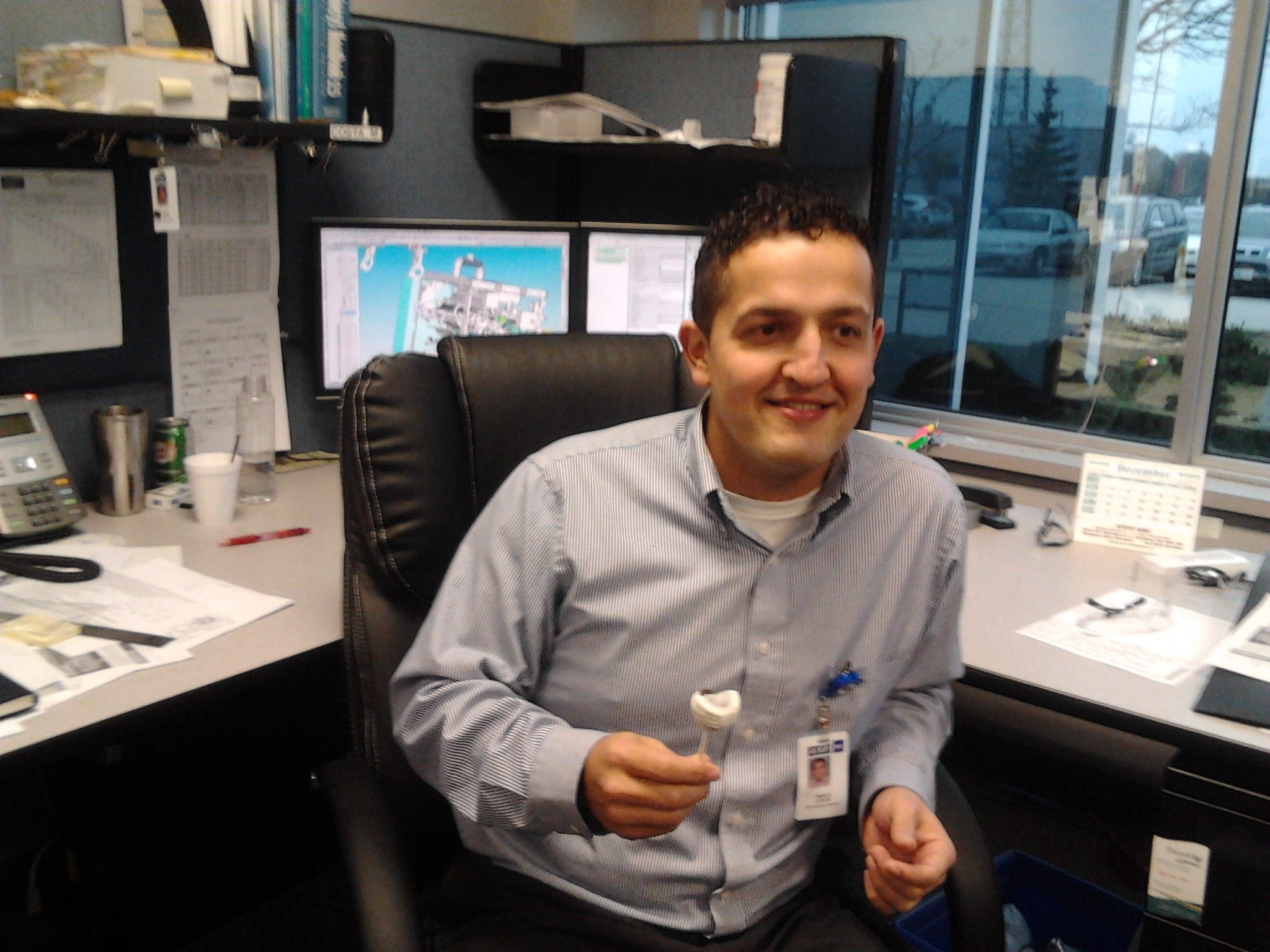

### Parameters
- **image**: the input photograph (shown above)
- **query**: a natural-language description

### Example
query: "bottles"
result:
[236,374,277,507]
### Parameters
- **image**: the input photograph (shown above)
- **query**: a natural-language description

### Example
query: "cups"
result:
[183,453,243,527]
[93,403,149,518]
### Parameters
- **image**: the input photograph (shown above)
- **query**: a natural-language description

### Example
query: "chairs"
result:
[330,336,1005,952]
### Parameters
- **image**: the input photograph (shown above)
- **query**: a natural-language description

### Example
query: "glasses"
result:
[1037,509,1073,545]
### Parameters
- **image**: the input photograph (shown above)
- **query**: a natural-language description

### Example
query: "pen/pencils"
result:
[218,527,309,546]
[905,418,942,455]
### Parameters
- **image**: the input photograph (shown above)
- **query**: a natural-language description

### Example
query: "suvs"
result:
[1071,194,1188,287]
[1230,204,1270,294]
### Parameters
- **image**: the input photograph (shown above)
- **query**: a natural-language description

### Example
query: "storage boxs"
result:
[895,849,1144,952]
[14,51,230,120]
[143,480,194,510]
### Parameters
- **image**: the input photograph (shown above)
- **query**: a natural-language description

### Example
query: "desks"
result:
[0,458,1270,952]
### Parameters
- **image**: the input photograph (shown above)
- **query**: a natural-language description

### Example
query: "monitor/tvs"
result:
[580,221,706,352]
[310,217,580,399]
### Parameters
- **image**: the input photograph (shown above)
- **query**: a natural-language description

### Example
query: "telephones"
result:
[0,394,89,539]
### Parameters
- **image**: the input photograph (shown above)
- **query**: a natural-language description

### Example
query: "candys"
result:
[691,687,743,731]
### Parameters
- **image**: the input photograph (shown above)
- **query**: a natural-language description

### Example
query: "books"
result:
[122,1,316,123]
[0,675,38,722]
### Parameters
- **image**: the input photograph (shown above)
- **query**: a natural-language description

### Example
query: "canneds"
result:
[151,416,194,488]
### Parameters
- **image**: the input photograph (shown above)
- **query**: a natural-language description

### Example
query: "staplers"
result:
[957,482,1014,530]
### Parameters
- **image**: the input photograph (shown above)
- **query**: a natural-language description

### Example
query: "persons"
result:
[807,757,829,787]
[388,174,970,952]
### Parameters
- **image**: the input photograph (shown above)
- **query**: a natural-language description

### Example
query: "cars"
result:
[1184,204,1206,274]
[889,191,955,238]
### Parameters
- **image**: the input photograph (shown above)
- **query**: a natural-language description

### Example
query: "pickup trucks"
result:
[974,206,1090,277]
